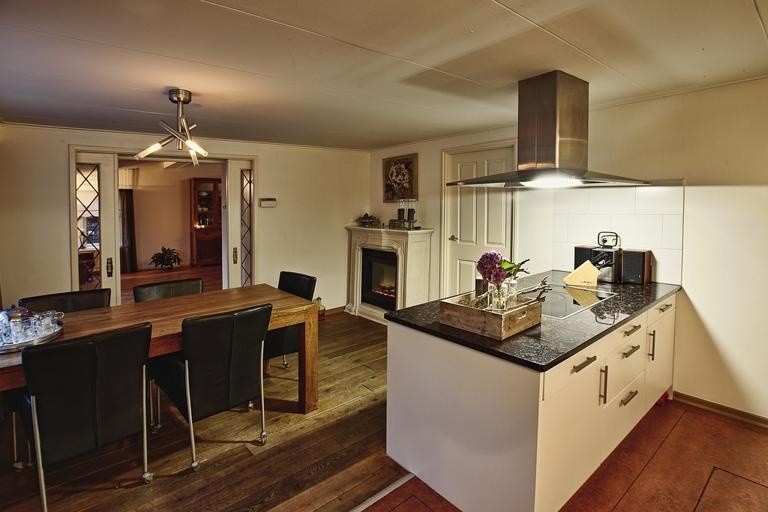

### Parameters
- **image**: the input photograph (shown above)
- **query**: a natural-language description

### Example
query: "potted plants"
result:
[148,246,181,270]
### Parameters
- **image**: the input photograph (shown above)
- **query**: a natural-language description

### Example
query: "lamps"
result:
[134,89,208,168]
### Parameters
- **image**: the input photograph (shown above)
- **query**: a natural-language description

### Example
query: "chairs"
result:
[147,302,273,473]
[133,277,203,429]
[18,287,112,467]
[12,320,153,511]
[265,271,318,371]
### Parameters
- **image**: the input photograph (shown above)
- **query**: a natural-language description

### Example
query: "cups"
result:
[0,309,65,345]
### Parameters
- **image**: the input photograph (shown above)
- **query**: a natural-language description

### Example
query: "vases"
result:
[487,280,518,314]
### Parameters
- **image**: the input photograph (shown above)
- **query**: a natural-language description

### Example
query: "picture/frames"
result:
[381,152,422,203]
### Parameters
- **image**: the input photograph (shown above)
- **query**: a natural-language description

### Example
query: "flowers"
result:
[477,253,530,307]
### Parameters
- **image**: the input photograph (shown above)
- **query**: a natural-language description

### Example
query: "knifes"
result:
[590,251,612,270]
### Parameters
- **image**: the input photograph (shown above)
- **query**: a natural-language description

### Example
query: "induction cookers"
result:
[515,282,619,320]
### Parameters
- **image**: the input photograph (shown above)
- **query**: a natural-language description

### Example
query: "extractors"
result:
[446,70,653,190]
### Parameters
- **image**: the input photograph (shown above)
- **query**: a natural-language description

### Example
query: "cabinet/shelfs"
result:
[78,251,96,283]
[536,292,676,512]
[190,177,223,266]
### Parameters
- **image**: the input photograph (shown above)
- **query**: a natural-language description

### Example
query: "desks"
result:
[2,282,319,416]
